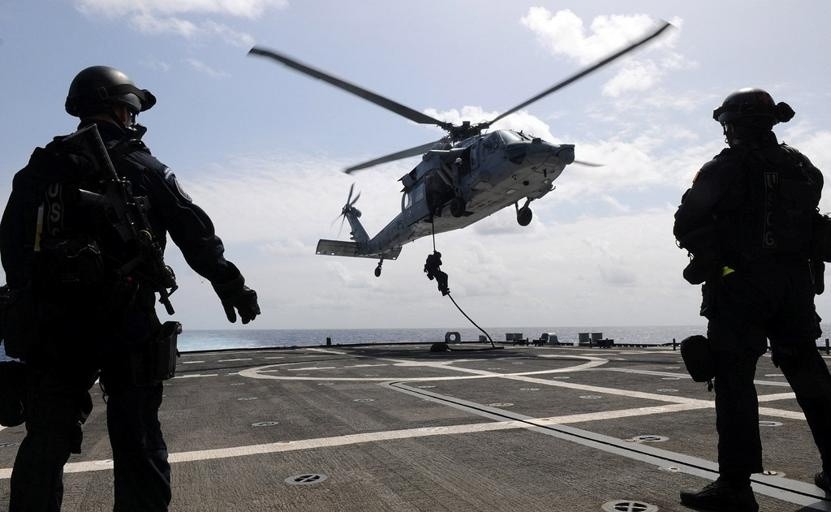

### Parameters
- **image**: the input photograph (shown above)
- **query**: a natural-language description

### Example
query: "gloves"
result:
[219,277,260,325]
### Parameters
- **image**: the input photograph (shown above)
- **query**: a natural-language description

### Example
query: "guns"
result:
[61,123,178,315]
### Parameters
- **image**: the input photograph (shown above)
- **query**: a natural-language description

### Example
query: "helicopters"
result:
[239,18,680,284]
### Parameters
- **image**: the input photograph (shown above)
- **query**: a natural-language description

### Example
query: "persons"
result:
[0,65,261,512]
[426,251,450,296]
[673,88,830,512]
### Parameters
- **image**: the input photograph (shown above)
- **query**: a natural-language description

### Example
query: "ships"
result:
[1,335,831,512]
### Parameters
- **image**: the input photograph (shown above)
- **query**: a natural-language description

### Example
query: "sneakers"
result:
[679,472,758,512]
[439,285,451,296]
[423,218,433,224]
[433,211,442,218]
[814,469,830,491]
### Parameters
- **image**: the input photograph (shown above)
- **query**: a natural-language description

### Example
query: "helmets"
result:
[65,65,144,117]
[712,89,776,121]
